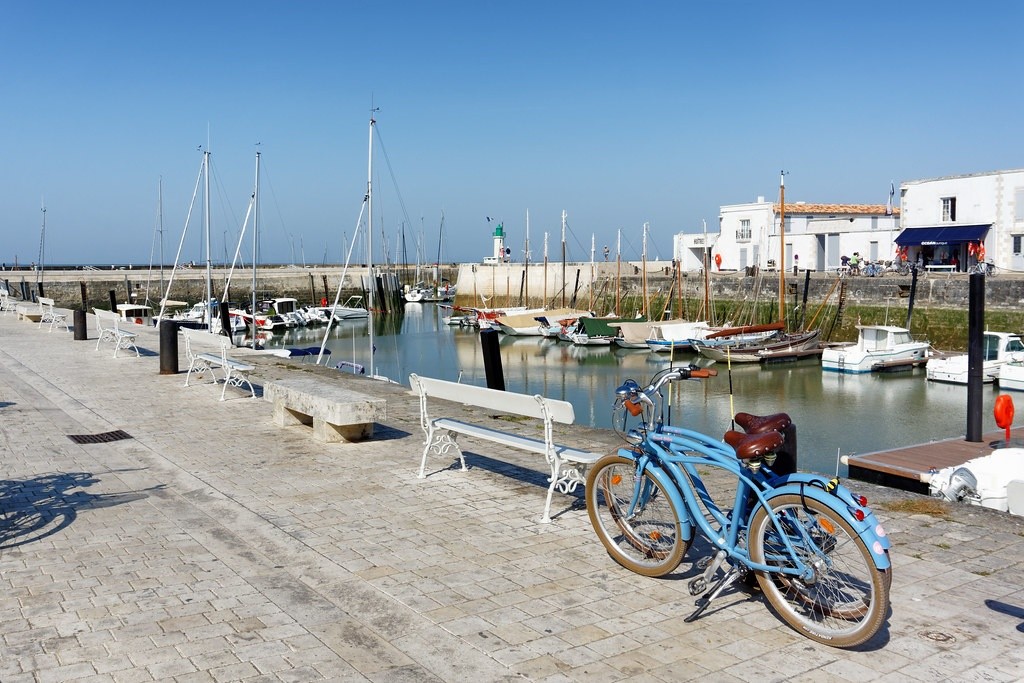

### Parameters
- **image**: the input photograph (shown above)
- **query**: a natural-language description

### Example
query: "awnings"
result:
[894,224,993,246]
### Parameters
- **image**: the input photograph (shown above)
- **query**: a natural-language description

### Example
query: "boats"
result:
[821,326,931,373]
[228,296,344,330]
[924,331,1024,384]
[316,295,368,319]
[91,303,155,329]
[998,356,1024,391]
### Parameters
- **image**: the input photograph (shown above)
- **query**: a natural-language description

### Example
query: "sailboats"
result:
[141,122,247,347]
[687,166,844,365]
[314,92,408,384]
[394,209,456,302]
[445,205,759,354]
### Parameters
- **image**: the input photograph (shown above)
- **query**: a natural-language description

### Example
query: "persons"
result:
[917,250,923,267]
[604,245,609,262]
[500,246,511,263]
[850,252,858,275]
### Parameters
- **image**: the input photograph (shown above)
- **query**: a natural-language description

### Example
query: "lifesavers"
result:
[474,324,479,328]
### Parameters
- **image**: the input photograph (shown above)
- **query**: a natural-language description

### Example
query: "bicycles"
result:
[585,361,894,648]
[859,260,884,277]
[967,259,1000,278]
[897,259,926,276]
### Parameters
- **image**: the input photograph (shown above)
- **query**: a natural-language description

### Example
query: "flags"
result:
[886,182,894,215]
[486,216,494,222]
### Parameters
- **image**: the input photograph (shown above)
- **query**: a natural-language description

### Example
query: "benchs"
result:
[92,306,141,358]
[824,266,850,279]
[179,326,256,402]
[36,295,69,333]
[408,372,606,523]
[15,304,42,322]
[0,289,18,316]
[263,378,387,443]
[924,265,957,280]
